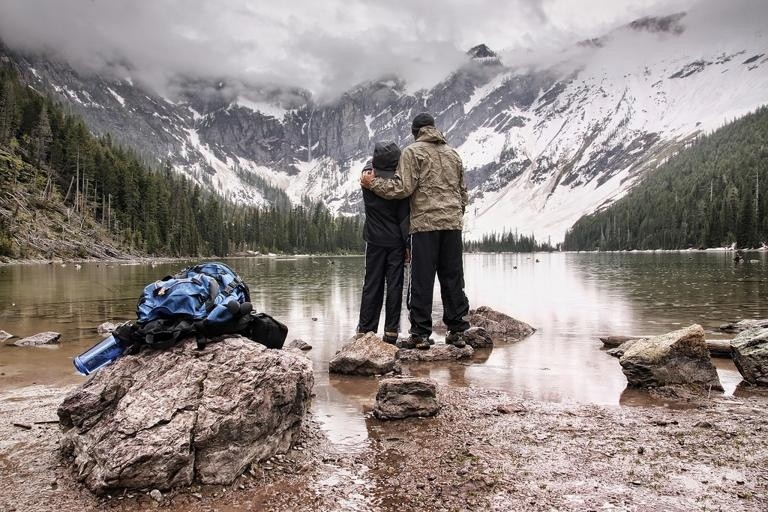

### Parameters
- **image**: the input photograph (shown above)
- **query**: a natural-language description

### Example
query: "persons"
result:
[354,140,412,344]
[360,113,469,349]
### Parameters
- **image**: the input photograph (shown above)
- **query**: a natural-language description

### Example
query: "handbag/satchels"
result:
[247,311,287,349]
[134,263,252,348]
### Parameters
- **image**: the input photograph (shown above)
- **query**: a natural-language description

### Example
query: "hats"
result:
[411,113,434,136]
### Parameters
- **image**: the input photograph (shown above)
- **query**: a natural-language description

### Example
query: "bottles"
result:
[74,334,124,376]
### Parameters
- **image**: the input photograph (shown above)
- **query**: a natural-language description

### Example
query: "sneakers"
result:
[406,334,431,349]
[383,329,398,343]
[356,323,375,335]
[445,329,466,347]
